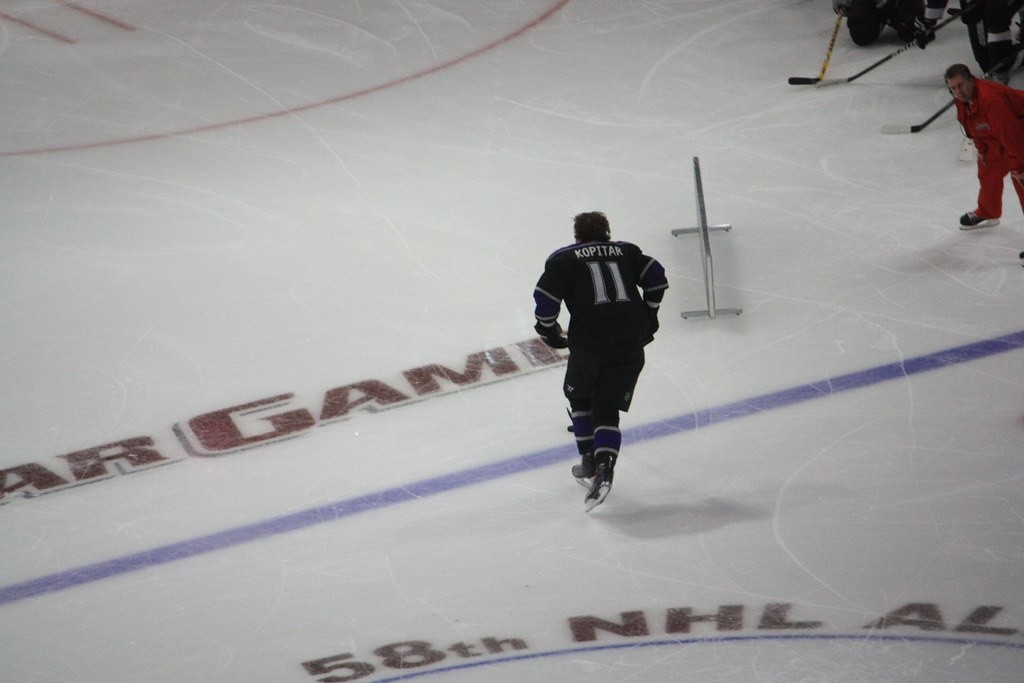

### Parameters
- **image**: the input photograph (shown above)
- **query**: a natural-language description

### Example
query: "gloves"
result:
[914,16,936,49]
[832,0,852,17]
[644,304,659,335]
[534,321,568,349]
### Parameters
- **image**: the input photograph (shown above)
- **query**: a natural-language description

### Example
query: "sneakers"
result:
[959,211,1000,230]
[572,459,596,488]
[584,455,614,512]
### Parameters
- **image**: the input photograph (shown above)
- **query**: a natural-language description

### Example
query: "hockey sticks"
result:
[788,5,961,89]
[880,61,1004,136]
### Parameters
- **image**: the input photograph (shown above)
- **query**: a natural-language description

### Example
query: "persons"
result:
[532,212,669,512]
[830,0,1024,265]
